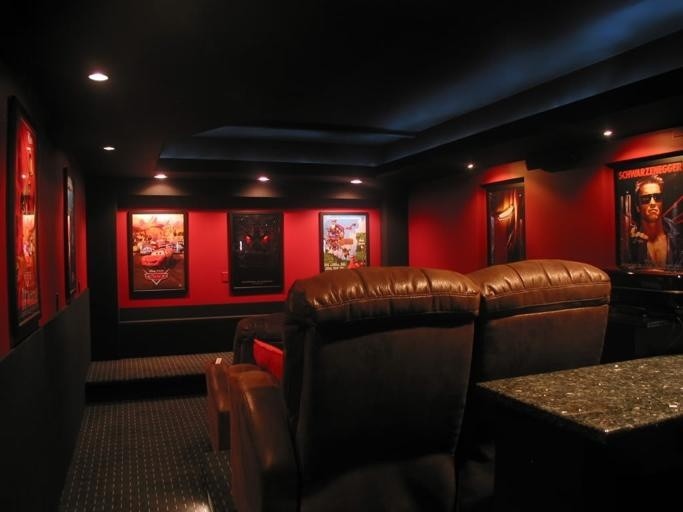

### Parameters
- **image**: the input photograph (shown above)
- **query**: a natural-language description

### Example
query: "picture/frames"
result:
[126,210,189,298]
[225,210,285,295]
[317,211,370,273]
[5,97,42,327]
[62,166,76,299]
[604,149,683,272]
[479,176,525,267]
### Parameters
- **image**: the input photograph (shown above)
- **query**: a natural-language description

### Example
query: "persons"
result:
[326,217,345,251]
[624,174,683,269]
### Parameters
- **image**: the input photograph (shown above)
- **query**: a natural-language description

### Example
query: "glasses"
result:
[635,193,666,205]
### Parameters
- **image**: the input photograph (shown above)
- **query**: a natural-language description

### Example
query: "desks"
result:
[477,339,682,512]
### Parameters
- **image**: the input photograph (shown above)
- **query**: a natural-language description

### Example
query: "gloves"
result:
[629,235,648,261]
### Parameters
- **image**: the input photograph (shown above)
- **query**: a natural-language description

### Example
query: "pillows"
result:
[251,337,286,376]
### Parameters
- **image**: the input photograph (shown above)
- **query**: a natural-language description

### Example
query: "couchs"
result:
[469,261,611,374]
[202,267,480,512]
[202,313,282,451]
